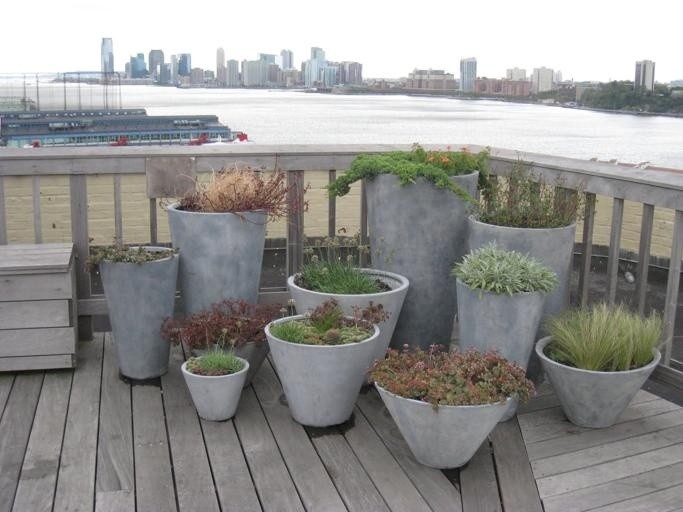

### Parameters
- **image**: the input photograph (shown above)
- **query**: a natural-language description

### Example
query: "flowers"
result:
[187,328,249,373]
[264,298,393,345]
[366,342,536,407]
[297,226,396,295]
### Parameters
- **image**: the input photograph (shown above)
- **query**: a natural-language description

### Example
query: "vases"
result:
[360,382,537,472]
[181,357,249,421]
[364,170,480,354]
[265,314,380,427]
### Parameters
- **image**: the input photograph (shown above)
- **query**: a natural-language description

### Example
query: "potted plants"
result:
[286,268,409,395]
[322,142,499,207]
[166,150,312,341]
[85,236,180,381]
[160,297,284,390]
[533,298,667,430]
[464,151,599,378]
[449,242,558,422]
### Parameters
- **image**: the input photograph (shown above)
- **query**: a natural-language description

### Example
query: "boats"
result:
[1,71,243,147]
